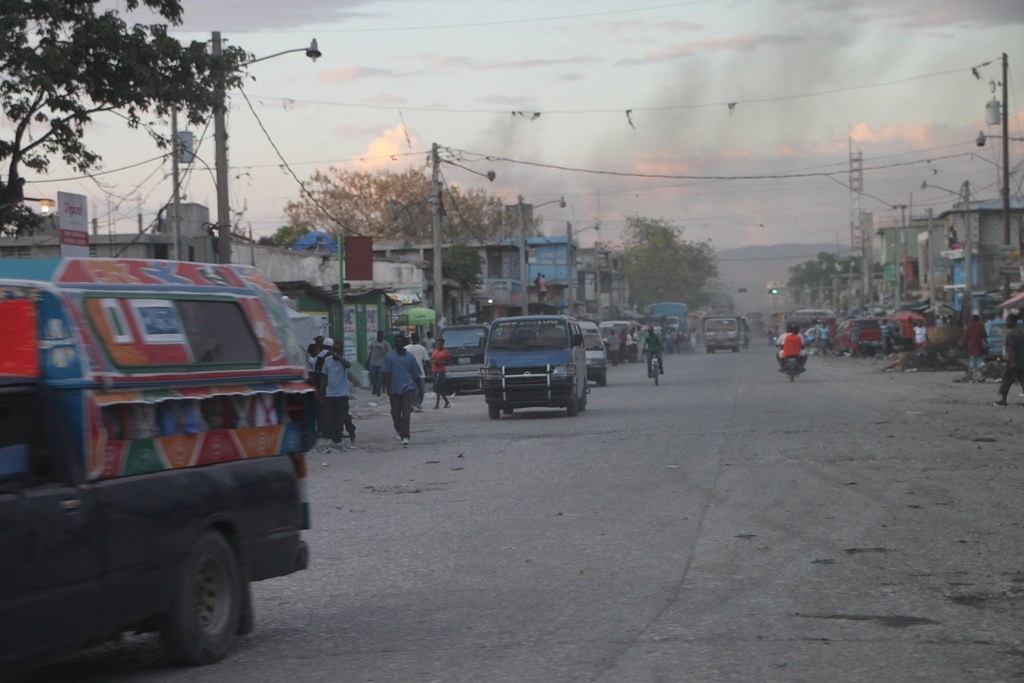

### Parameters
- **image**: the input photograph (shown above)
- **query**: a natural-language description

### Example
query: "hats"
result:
[647,326,653,331]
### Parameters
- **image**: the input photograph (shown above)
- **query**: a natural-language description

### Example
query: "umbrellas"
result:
[392,305,436,337]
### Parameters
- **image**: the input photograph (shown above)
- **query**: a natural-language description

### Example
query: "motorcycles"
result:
[773,336,808,380]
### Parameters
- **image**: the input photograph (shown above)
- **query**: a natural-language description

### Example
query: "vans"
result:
[573,320,610,387]
[598,302,688,366]
[597,320,647,363]
[434,319,492,396]
[765,304,1024,378]
[479,313,589,420]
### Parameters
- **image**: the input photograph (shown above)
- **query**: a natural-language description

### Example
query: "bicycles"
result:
[641,347,665,386]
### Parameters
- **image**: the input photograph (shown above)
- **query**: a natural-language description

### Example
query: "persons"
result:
[381,336,424,445]
[903,316,927,351]
[993,314,1024,408]
[430,339,452,409]
[964,315,988,369]
[366,330,392,396]
[606,326,644,366]
[306,336,356,447]
[400,332,435,413]
[805,319,860,357]
[534,272,548,302]
[879,319,891,352]
[664,327,699,354]
[436,313,447,329]
[935,314,952,325]
[776,324,808,372]
[643,326,664,378]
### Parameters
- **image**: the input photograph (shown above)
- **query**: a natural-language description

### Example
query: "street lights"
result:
[919,177,971,323]
[212,31,323,265]
[517,192,567,315]
[565,220,601,317]
[431,141,497,341]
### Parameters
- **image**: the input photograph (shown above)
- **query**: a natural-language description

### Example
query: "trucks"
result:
[703,315,750,354]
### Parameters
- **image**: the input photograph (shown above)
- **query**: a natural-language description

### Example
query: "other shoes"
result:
[331,442,337,447]
[396,432,401,439]
[403,437,408,445]
[350,433,355,441]
[993,400,1006,408]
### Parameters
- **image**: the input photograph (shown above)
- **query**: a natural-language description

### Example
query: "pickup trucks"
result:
[0,370,314,683]
